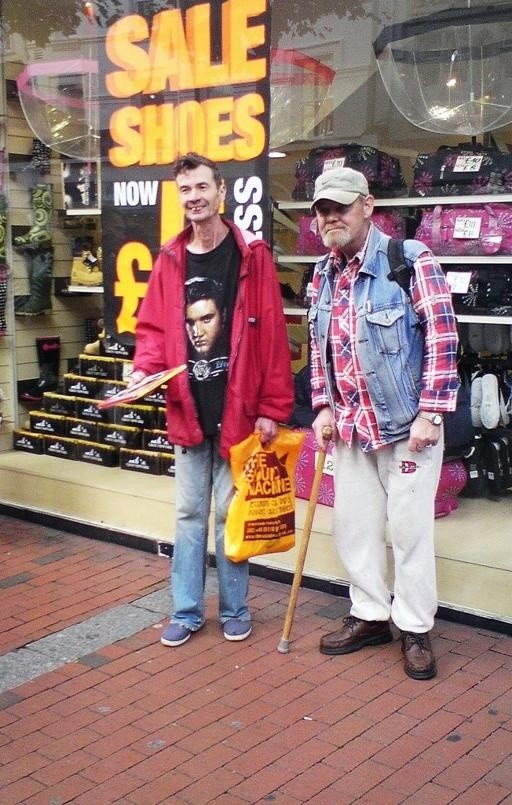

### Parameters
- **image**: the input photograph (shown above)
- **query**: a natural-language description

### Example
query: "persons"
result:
[309,168,459,679]
[183,276,226,355]
[127,153,296,646]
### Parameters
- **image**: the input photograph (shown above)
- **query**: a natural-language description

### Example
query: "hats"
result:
[310,167,369,212]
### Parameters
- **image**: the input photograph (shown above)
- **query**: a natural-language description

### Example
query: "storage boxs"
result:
[12,338,177,478]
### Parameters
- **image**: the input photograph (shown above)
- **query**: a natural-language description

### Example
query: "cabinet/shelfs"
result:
[64,134,512,326]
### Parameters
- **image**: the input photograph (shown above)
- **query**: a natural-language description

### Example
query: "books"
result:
[97,365,188,411]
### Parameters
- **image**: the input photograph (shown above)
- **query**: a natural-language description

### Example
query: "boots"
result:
[20,137,52,176]
[84,328,105,355]
[15,246,55,318]
[72,258,103,287]
[23,336,61,400]
[13,184,54,245]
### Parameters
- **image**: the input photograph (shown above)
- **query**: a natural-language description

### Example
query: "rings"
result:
[425,444,432,448]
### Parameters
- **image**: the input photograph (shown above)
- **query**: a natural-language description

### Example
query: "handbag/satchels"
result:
[452,274,511,316]
[296,208,406,257]
[443,379,473,454]
[292,142,408,201]
[410,141,511,198]
[411,204,511,256]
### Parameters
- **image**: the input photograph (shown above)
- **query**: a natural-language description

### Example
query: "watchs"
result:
[417,411,444,425]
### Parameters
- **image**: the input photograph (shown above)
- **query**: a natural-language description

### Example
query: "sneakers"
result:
[161,623,192,647]
[460,364,512,496]
[224,618,250,642]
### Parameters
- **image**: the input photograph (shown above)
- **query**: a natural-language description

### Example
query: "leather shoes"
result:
[400,630,438,680]
[320,615,394,655]
